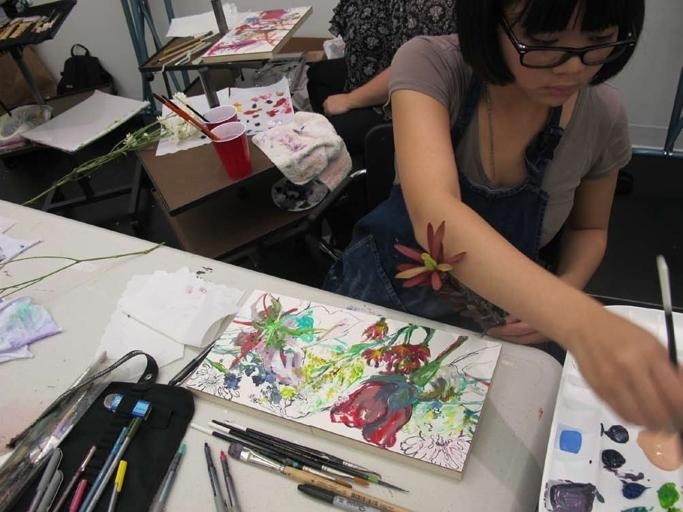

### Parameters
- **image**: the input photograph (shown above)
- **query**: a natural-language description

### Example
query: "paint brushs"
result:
[191,417,413,512]
[57,349,107,408]
[151,30,215,66]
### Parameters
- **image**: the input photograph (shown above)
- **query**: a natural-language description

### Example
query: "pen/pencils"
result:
[148,93,217,141]
[167,340,217,386]
[654,254,679,362]
[29,416,147,511]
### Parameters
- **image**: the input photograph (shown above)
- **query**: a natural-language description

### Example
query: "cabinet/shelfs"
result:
[0,0,312,262]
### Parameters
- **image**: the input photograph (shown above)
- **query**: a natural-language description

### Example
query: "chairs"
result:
[304,124,396,289]
[534,147,683,363]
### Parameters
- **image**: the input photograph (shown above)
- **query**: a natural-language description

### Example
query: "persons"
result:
[339,0,683,433]
[306,0,461,138]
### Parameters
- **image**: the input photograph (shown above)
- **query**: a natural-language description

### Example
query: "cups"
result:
[201,104,238,131]
[207,121,253,182]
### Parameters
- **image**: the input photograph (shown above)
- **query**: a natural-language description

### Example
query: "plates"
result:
[270,174,328,212]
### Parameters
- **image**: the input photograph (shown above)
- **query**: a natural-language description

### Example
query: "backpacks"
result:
[57,44,118,95]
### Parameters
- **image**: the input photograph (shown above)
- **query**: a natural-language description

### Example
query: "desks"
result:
[0,201,565,511]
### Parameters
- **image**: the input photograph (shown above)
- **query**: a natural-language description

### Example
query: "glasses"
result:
[499,13,637,69]
